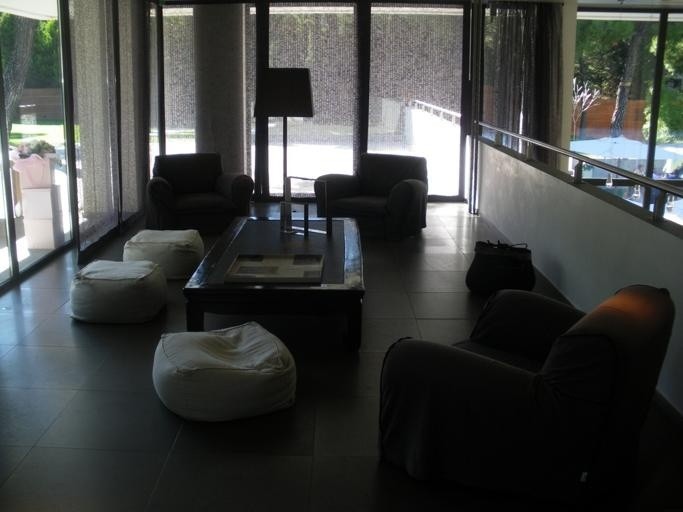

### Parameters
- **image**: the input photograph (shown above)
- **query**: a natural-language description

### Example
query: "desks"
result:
[180,216,366,370]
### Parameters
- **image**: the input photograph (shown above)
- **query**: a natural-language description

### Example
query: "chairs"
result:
[375,281,677,512]
[314,151,429,242]
[143,151,255,231]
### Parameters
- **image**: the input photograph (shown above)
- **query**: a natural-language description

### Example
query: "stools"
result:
[148,320,298,423]
[122,227,206,282]
[68,256,168,325]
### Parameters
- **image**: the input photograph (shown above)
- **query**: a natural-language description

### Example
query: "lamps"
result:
[252,67,316,221]
